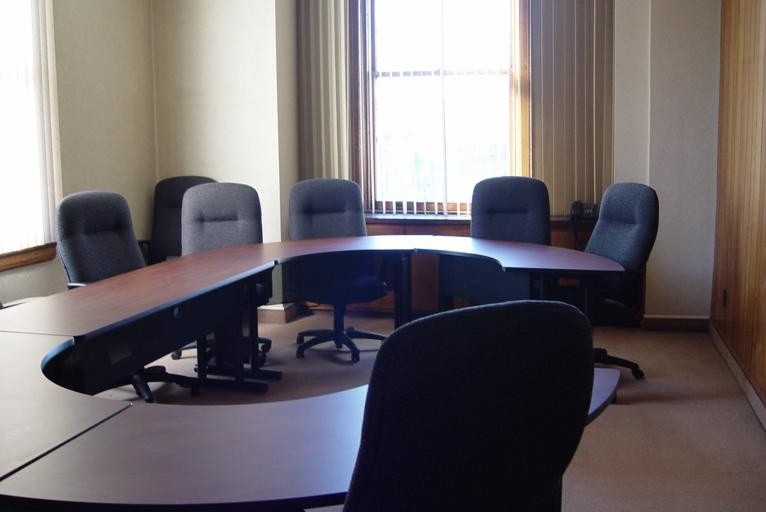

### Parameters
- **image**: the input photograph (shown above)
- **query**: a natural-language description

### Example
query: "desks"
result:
[0,231,630,512]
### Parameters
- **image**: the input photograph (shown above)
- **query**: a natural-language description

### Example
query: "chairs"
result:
[279,174,394,366]
[327,300,590,512]
[555,178,661,385]
[51,186,208,401]
[144,174,226,363]
[165,179,281,386]
[470,173,557,249]
